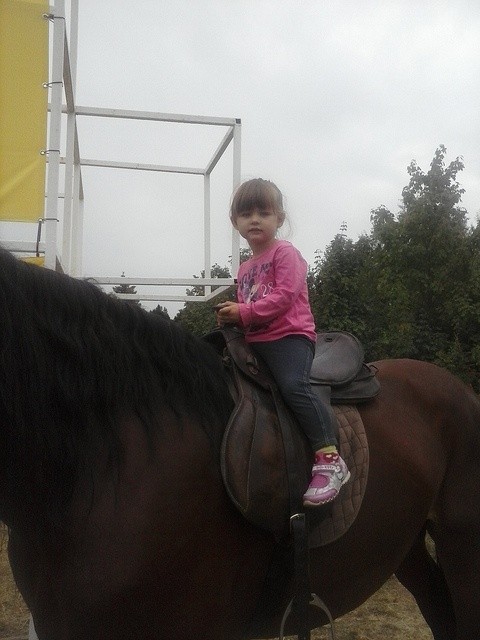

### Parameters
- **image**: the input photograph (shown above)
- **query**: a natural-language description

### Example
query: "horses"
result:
[0,249,480,640]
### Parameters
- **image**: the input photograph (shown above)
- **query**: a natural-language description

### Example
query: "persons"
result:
[214,179,352,507]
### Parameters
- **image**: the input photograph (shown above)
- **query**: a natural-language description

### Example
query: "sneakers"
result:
[301,454,353,509]
